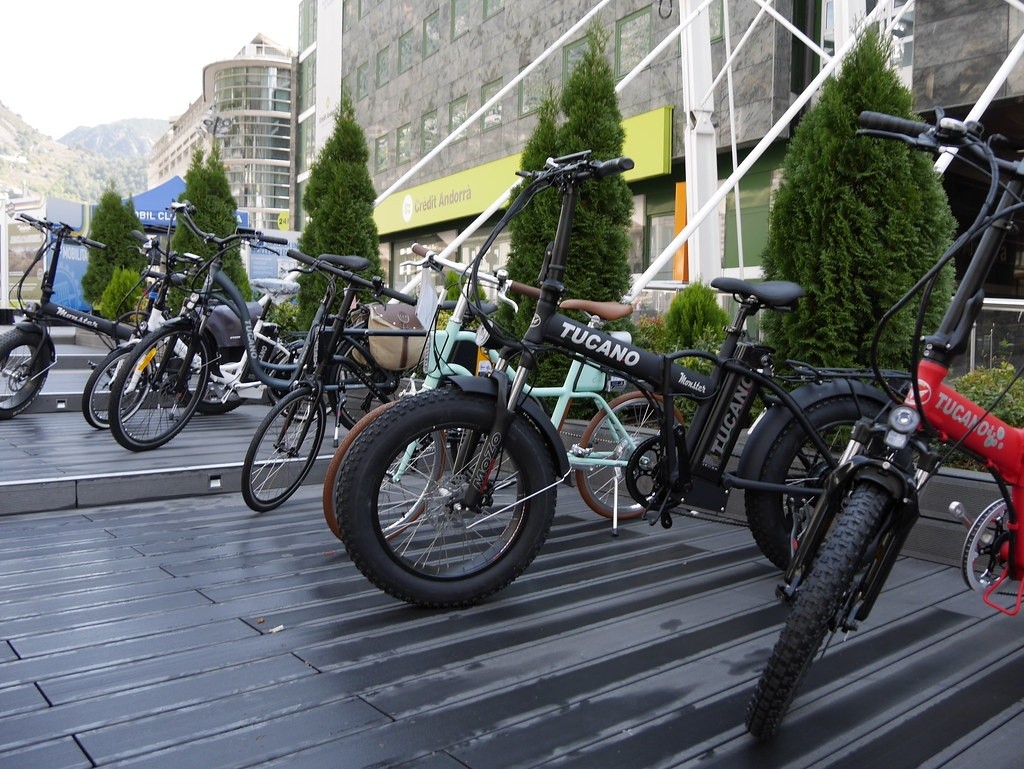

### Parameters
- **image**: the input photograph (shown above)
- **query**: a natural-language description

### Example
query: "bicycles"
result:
[0,199,549,452]
[333,150,911,609]
[242,248,548,513]
[322,243,686,542]
[744,109,1024,740]
